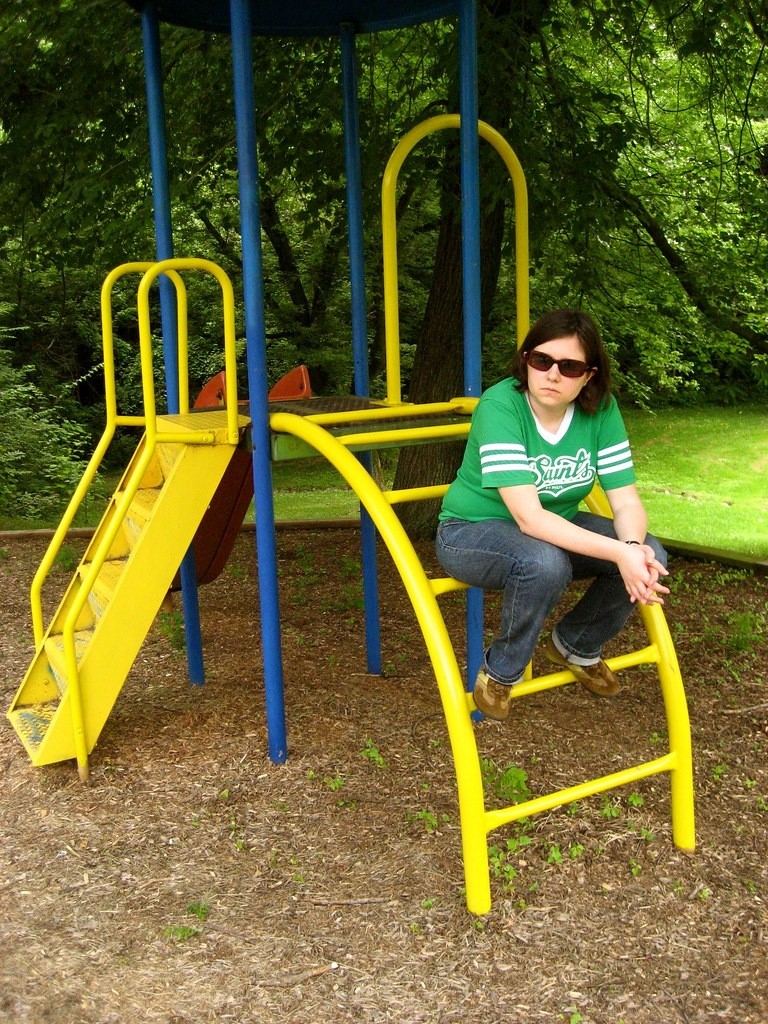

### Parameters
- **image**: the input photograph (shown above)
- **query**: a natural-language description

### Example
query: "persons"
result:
[437,309,670,721]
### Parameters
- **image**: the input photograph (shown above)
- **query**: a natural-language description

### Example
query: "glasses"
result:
[526,348,590,377]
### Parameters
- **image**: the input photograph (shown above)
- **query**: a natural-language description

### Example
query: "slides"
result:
[168,364,312,593]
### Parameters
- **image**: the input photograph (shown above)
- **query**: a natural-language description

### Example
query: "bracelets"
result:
[625,541,640,546]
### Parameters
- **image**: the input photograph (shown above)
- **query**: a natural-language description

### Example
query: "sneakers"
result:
[472,658,511,721]
[540,631,622,699]
